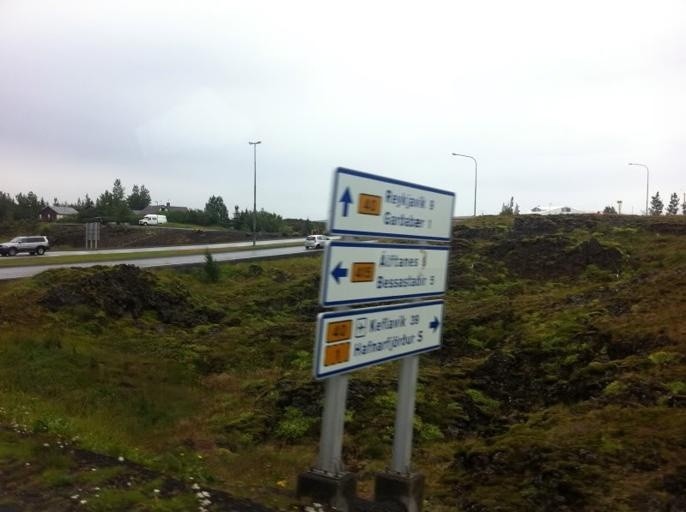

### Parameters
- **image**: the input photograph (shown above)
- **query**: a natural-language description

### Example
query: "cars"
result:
[0,235,50,256]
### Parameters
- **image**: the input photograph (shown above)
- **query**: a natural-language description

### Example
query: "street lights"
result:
[452,153,478,216]
[628,163,649,215]
[248,139,261,245]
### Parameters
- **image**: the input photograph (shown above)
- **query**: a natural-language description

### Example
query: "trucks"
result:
[139,214,167,226]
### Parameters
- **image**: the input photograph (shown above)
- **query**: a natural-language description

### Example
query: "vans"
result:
[304,235,330,249]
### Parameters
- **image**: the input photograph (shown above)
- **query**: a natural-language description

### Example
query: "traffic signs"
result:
[314,167,456,381]
